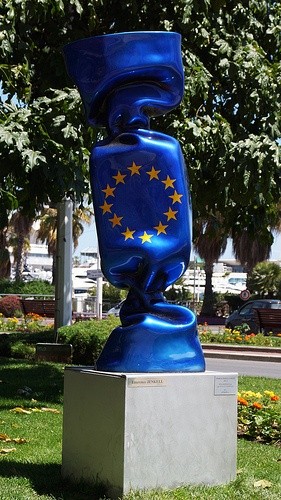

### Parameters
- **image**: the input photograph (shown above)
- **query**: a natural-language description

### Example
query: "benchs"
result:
[17,299,56,317]
[251,307,281,335]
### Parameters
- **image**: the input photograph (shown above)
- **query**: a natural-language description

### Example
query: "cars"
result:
[224,299,281,330]
[106,299,127,316]
